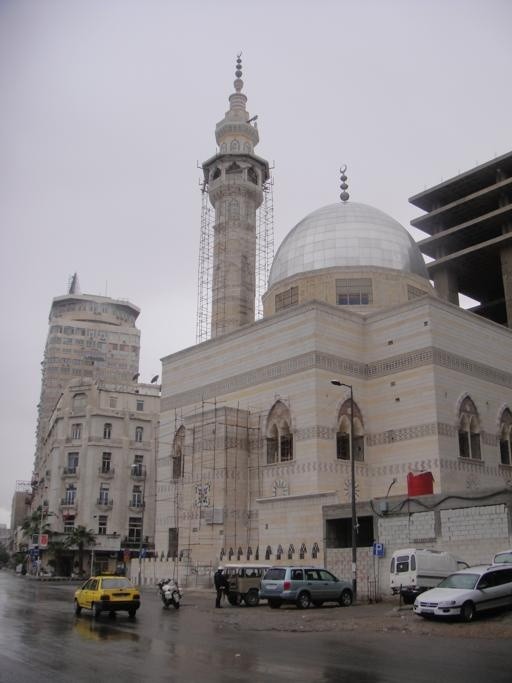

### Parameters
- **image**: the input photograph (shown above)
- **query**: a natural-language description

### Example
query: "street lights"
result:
[330,379,359,601]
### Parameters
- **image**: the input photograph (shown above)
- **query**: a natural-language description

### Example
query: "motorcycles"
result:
[158,578,181,608]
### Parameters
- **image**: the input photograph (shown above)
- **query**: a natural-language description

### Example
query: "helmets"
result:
[217,566,224,569]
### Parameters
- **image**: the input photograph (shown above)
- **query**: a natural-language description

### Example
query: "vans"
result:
[390,547,472,603]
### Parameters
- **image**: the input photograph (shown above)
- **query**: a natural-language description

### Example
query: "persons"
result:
[214,566,225,608]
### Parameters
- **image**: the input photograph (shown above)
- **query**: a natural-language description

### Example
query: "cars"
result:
[74,575,141,617]
[412,552,511,622]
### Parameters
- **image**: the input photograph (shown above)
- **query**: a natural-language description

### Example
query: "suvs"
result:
[223,564,273,606]
[260,565,355,608]
